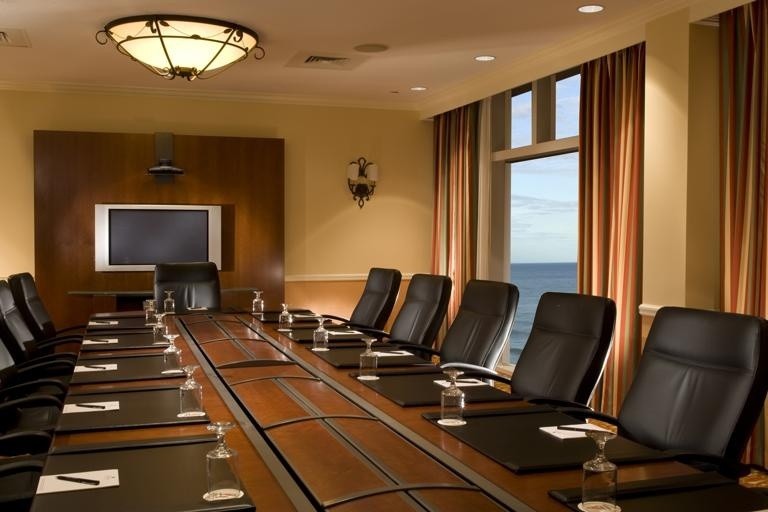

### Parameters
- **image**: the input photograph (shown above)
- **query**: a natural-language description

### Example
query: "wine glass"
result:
[580,429,617,511]
[145,299,204,414]
[205,422,241,497]
[439,368,466,423]
[279,303,293,330]
[162,290,175,313]
[311,317,330,349]
[359,337,379,380]
[252,290,265,314]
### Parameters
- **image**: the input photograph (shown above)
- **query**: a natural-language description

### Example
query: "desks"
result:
[30,307,768,511]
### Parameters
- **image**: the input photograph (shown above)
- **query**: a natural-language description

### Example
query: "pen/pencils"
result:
[380,351,404,354]
[57,476,99,485]
[85,366,106,369]
[557,426,615,435]
[447,380,478,383]
[76,404,105,409]
[91,322,109,342]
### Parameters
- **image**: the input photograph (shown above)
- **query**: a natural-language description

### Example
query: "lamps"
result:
[346,157,378,211]
[95,14,265,82]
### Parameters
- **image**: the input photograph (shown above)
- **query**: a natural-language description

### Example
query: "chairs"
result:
[0,269,89,511]
[320,268,403,337]
[551,305,768,461]
[392,279,521,389]
[458,292,616,413]
[154,262,222,313]
[353,274,452,364]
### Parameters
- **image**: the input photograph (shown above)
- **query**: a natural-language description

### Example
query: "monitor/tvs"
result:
[95,204,222,272]
